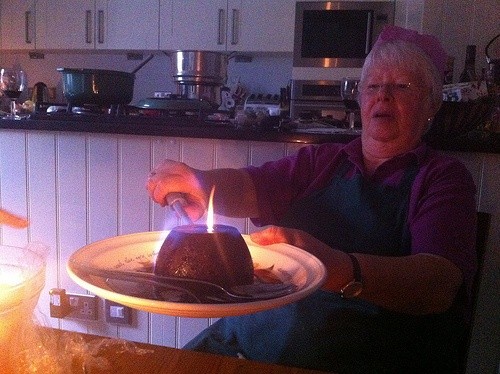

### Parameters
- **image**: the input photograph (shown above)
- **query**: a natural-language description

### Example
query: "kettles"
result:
[31,82,50,109]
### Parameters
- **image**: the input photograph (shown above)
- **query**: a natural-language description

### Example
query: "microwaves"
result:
[292,1,395,69]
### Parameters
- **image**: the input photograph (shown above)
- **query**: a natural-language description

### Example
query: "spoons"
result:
[75,262,299,298]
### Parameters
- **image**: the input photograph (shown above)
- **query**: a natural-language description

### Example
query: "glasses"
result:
[361,80,412,94]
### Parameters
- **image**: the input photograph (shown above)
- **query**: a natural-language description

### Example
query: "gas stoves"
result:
[30,108,235,127]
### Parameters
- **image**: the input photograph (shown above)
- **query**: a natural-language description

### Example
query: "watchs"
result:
[334,254,363,300]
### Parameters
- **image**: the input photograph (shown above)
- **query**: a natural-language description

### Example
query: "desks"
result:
[0,324,333,374]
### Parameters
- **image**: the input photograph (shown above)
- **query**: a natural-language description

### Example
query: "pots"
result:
[57,52,155,105]
[159,49,239,107]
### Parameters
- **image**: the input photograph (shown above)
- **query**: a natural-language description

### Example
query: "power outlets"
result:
[64,293,99,323]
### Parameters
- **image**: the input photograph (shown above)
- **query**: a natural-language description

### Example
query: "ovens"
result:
[288,79,360,129]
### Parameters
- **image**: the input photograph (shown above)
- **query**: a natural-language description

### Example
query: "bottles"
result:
[459,44,476,83]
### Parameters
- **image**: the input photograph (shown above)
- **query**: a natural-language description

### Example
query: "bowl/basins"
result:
[0,243,47,374]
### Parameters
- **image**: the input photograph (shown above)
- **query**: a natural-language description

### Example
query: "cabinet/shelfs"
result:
[392,0,500,71]
[0,0,298,58]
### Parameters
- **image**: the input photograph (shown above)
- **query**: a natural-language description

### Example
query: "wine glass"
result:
[339,77,362,135]
[0,68,30,121]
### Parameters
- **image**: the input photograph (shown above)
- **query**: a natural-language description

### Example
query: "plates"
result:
[67,230,328,318]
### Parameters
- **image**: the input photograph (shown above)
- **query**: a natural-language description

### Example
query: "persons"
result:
[145,25,479,374]
